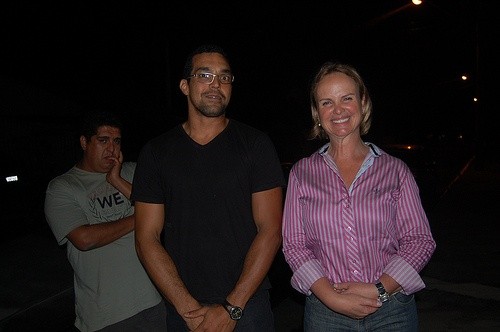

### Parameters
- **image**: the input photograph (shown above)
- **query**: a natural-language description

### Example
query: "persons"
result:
[45,109,168,332]
[129,44,287,332]
[282,62,436,332]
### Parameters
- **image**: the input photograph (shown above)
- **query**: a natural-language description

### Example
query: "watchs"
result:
[221,298,243,321]
[375,280,390,303]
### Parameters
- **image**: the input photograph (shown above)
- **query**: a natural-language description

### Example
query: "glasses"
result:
[187,72,235,84]
